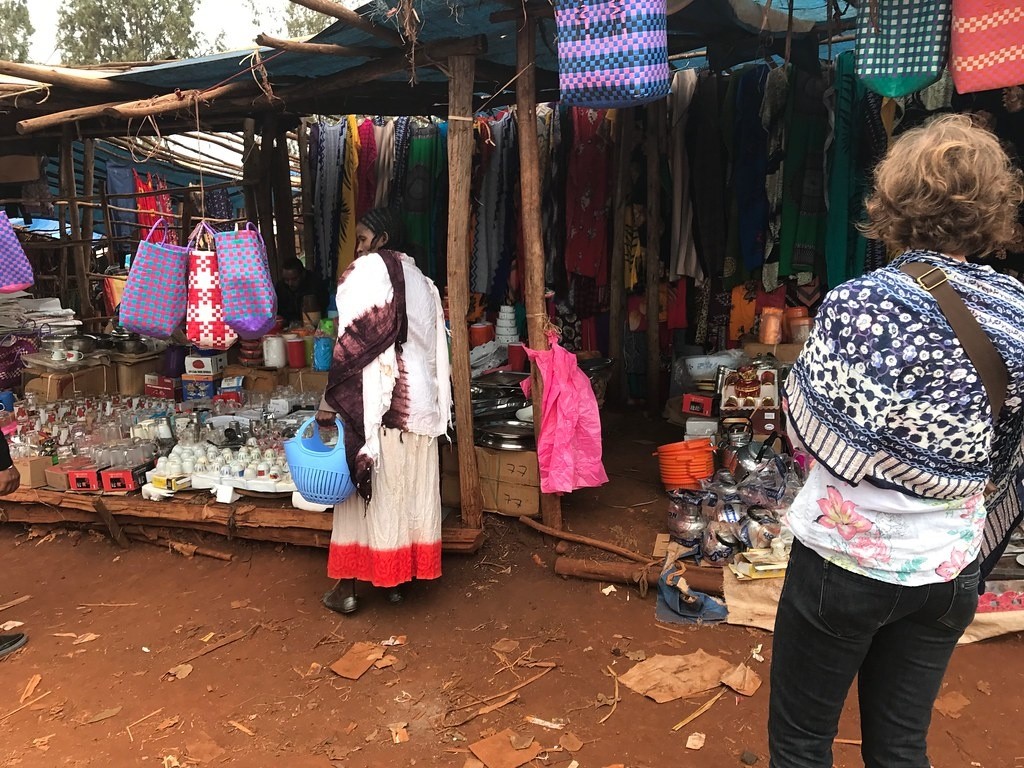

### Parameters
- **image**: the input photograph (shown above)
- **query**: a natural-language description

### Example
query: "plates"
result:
[469,341,501,367]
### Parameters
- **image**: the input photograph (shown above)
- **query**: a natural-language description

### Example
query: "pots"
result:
[113,333,148,355]
[89,331,113,349]
[63,330,96,353]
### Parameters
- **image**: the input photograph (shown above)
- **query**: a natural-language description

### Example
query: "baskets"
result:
[282,414,357,506]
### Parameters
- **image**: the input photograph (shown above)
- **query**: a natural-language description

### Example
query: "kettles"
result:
[749,351,793,379]
[314,337,333,371]
[665,416,805,566]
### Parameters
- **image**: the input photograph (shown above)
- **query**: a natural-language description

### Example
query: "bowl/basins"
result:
[683,354,739,381]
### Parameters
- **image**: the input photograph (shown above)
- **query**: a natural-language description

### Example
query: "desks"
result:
[0,484,483,553]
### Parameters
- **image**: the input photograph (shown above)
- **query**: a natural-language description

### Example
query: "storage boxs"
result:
[442,445,540,516]
[13,353,329,492]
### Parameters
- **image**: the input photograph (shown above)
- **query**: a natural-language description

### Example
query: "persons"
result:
[765,111,1024,767]
[314,208,455,615]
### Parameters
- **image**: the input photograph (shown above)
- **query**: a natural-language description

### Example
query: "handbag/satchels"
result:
[119,218,279,351]
[554,0,672,110]
[0,210,36,295]
[853,0,1024,98]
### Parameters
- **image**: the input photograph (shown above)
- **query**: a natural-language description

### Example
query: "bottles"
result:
[304,336,313,365]
[783,307,813,343]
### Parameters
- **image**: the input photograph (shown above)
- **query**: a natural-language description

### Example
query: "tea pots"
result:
[302,294,321,325]
[327,295,338,319]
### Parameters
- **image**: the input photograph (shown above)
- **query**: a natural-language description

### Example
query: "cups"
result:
[50,348,67,360]
[507,343,528,371]
[470,317,495,345]
[175,418,190,433]
[263,337,286,368]
[287,339,306,368]
[66,350,84,361]
[176,384,320,415]
[157,437,294,484]
[0,392,176,469]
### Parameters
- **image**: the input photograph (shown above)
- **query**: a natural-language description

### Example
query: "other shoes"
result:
[0,633,30,657]
[380,584,406,606]
[322,577,373,615]
